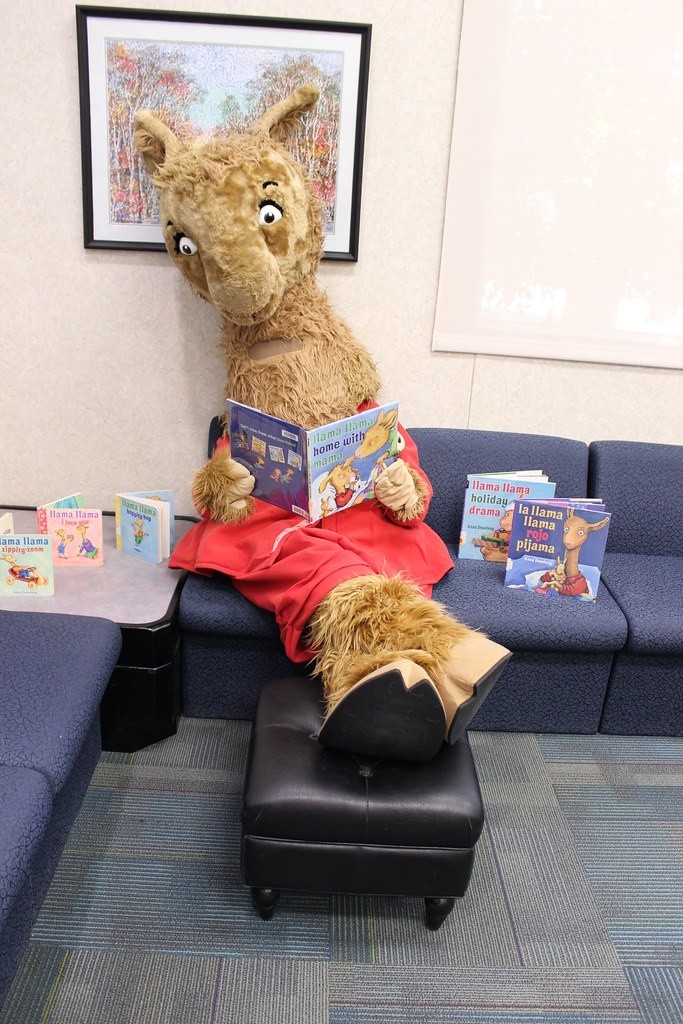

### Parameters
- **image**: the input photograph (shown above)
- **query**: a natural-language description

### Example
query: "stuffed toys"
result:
[133,85,510,762]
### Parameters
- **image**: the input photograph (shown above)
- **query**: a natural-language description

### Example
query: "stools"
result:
[238,676,485,931]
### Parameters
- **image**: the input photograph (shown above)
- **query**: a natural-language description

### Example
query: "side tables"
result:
[0,505,200,755]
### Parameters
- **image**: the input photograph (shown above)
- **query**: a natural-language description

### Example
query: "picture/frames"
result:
[76,4,373,263]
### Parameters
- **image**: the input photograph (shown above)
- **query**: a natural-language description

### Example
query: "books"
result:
[36,492,104,568]
[503,498,611,603]
[0,512,55,596]
[228,399,398,525]
[115,489,176,565]
[457,469,556,564]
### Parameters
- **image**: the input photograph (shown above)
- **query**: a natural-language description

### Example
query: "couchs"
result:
[180,414,682,739]
[1,610,121,930]
